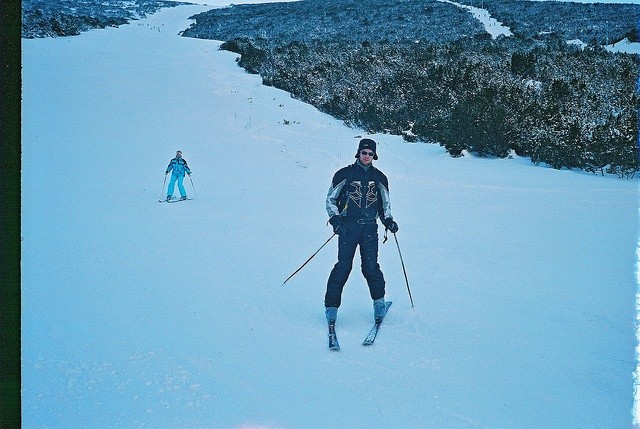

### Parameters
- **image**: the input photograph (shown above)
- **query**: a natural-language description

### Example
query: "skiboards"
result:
[158,196,193,203]
[328,302,393,350]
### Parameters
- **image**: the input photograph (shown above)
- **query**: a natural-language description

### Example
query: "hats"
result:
[176,151,182,158]
[355,139,378,160]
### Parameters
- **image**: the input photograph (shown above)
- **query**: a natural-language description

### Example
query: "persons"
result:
[164,151,191,203]
[324,139,398,327]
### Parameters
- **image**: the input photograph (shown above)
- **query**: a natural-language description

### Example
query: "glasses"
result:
[360,151,373,156]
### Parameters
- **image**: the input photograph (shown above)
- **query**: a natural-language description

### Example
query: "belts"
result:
[344,217,377,225]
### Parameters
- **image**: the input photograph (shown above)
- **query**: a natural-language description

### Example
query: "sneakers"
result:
[326,307,337,320]
[179,196,186,201]
[373,297,385,318]
[165,195,171,202]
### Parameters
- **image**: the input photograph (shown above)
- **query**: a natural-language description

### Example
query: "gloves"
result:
[165,171,170,175]
[326,215,344,235]
[188,173,192,177]
[382,218,398,233]
